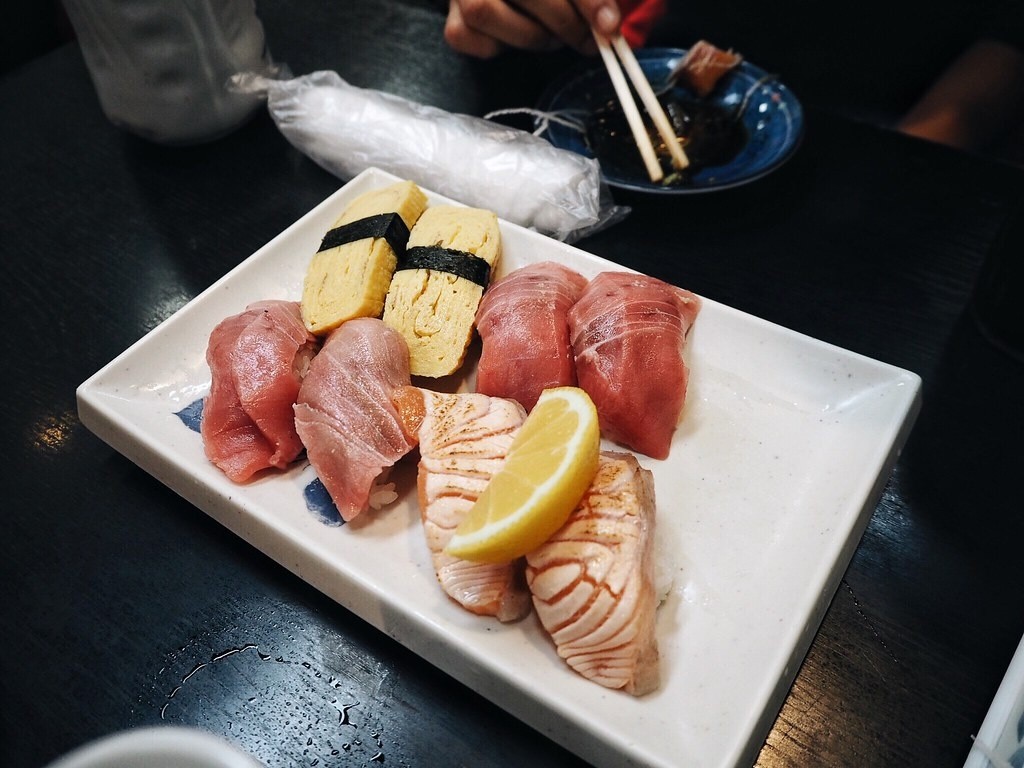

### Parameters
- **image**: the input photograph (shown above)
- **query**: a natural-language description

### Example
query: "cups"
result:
[63,0,278,146]
[36,724,263,768]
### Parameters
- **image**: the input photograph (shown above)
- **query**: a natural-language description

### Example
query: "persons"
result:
[442,0,1024,153]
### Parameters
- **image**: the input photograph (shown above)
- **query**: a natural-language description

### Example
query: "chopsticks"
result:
[592,20,691,182]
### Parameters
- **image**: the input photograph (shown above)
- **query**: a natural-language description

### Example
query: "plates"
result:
[70,166,923,768]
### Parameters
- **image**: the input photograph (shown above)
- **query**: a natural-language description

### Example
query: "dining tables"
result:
[1,1,1024,768]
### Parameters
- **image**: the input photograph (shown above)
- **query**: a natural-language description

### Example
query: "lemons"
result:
[443,386,601,565]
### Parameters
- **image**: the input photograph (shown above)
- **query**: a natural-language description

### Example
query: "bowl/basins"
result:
[549,49,804,195]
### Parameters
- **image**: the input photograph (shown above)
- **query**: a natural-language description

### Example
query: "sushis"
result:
[198,260,702,696]
